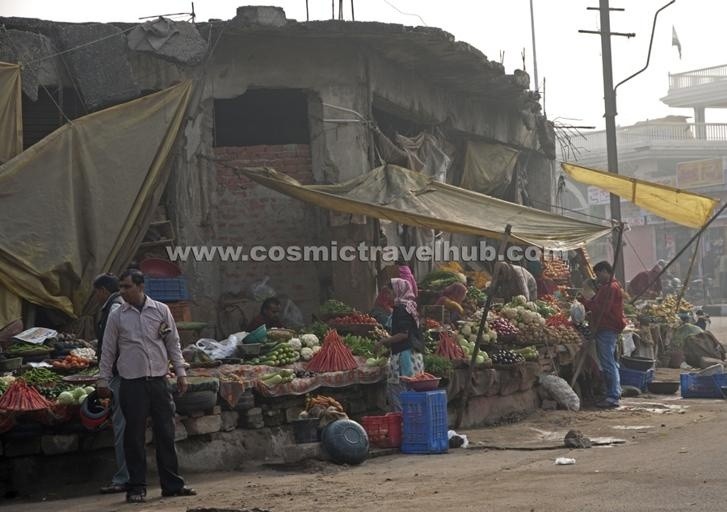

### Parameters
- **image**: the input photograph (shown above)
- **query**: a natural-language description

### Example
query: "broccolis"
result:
[286,333,322,361]
[500,293,545,331]
[461,320,498,345]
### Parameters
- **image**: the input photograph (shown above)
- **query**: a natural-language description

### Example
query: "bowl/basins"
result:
[0,357,23,370]
[0,318,25,339]
[318,418,370,465]
[235,324,294,359]
[408,376,443,392]
[647,378,682,396]
[620,352,656,372]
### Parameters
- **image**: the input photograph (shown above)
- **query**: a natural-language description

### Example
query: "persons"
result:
[247,298,284,332]
[93,271,130,494]
[98,267,196,502]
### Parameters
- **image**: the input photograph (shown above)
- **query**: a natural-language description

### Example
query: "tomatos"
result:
[334,314,379,328]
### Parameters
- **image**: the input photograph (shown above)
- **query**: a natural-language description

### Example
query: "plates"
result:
[46,365,90,376]
[61,374,100,386]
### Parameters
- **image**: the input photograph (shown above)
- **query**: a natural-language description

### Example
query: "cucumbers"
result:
[244,342,299,365]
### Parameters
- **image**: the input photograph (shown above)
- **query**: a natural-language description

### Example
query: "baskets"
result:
[397,388,449,455]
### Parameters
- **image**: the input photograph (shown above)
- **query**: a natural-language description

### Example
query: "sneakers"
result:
[125,492,145,502]
[594,398,619,407]
[170,485,198,496]
[164,302,192,323]
[98,484,127,493]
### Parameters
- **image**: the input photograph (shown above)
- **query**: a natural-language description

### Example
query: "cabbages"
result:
[56,385,95,405]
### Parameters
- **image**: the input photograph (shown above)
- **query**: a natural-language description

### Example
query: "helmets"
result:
[78,389,116,432]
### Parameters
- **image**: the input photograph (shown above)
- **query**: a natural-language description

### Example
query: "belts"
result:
[118,375,166,382]
